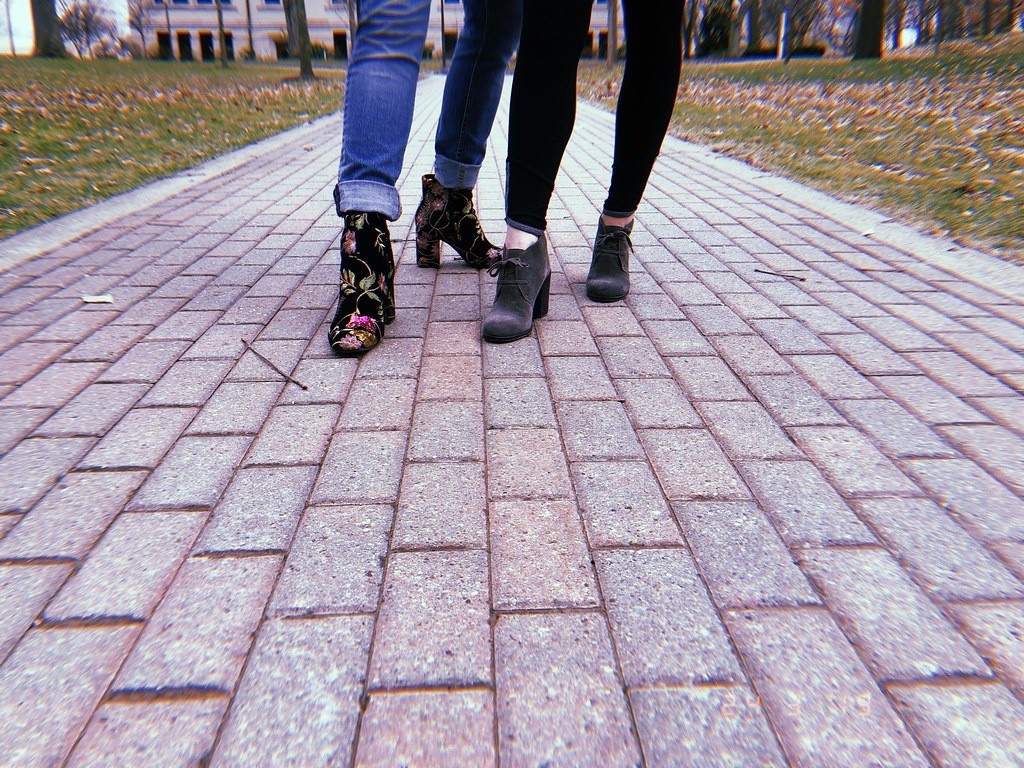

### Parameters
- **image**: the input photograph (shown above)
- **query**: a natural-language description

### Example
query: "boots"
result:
[327,210,396,356]
[416,174,503,269]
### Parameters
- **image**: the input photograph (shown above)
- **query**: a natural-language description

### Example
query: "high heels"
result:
[483,232,551,343]
[585,214,634,302]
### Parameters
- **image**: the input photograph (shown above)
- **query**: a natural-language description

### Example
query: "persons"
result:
[482,0,686,342]
[329,0,523,357]
